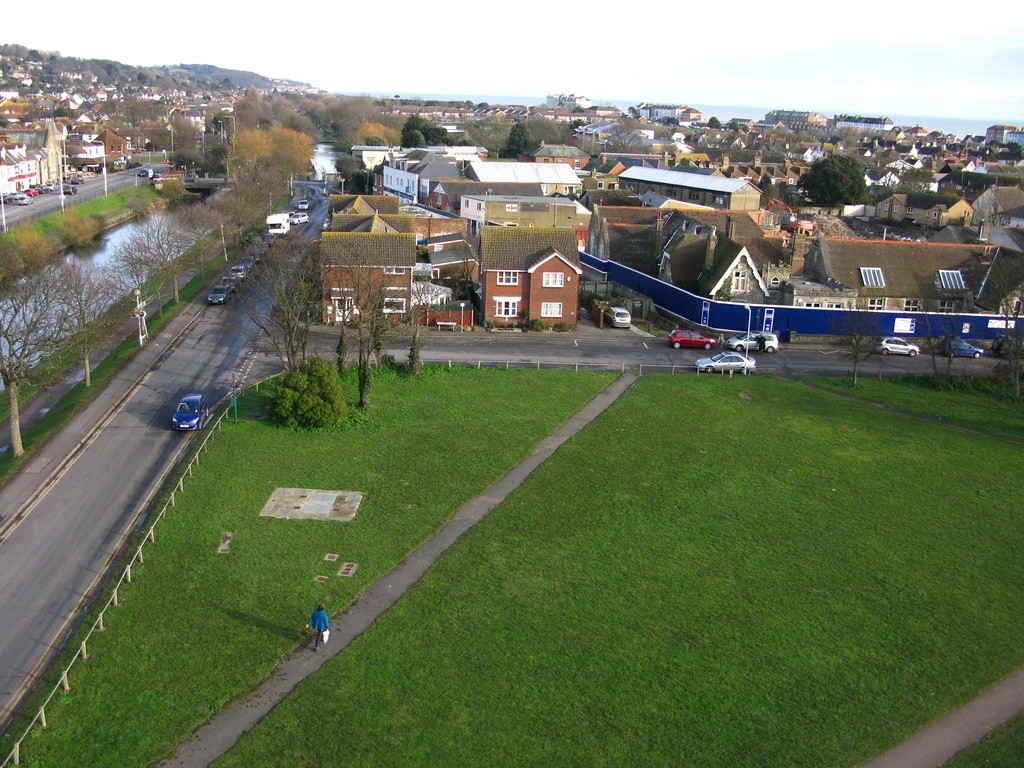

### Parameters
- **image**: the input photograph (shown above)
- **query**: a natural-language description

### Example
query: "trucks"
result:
[265,213,290,237]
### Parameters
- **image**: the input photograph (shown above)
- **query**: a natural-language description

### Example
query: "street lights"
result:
[133,288,143,347]
[217,120,223,143]
[743,304,751,375]
[93,140,107,197]
[224,115,235,152]
[167,129,173,154]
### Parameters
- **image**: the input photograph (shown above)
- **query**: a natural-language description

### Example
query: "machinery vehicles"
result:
[765,198,814,239]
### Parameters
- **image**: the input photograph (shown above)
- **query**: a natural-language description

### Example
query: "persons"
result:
[756,333,766,356]
[718,333,725,350]
[305,603,330,654]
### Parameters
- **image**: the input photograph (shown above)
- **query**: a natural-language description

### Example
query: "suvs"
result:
[668,328,716,350]
[726,331,779,354]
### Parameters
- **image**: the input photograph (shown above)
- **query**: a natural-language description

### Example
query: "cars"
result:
[63,185,78,196]
[17,195,34,206]
[71,177,86,184]
[220,233,276,293]
[875,337,920,357]
[0,182,58,204]
[695,350,757,374]
[321,219,330,231]
[172,394,205,432]
[938,337,984,359]
[288,211,296,218]
[138,169,163,182]
[289,213,310,225]
[298,199,310,210]
[183,174,200,183]
[207,284,232,305]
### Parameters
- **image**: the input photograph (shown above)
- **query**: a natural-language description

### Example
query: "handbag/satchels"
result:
[323,629,330,643]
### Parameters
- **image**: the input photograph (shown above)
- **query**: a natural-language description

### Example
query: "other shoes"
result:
[316,648,321,652]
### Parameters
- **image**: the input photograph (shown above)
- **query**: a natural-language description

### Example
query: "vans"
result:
[604,306,632,329]
